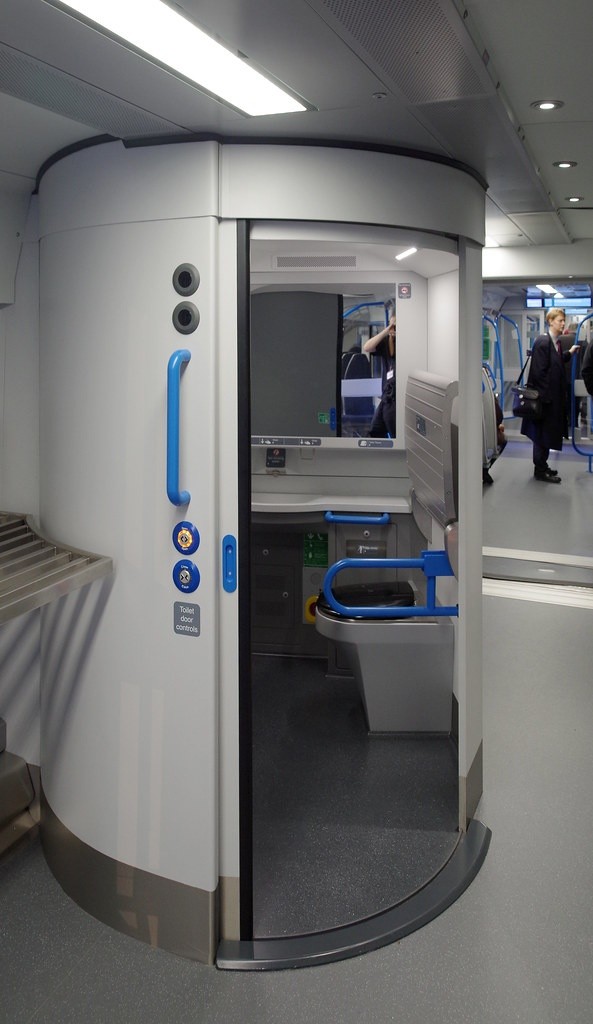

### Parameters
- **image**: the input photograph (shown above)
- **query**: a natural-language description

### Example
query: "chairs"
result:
[341,351,374,424]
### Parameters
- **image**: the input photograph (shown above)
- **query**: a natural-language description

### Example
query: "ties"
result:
[556,340,564,369]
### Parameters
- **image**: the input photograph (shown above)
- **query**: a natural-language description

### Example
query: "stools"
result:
[315,579,452,737]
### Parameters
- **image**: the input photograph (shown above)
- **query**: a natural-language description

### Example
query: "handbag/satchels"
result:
[511,384,542,418]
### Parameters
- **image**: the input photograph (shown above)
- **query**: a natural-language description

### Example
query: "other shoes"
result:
[548,468,558,474]
[483,468,494,484]
[535,471,561,483]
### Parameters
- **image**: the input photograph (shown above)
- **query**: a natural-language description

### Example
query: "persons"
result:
[483,397,507,486]
[364,316,396,439]
[521,308,581,483]
